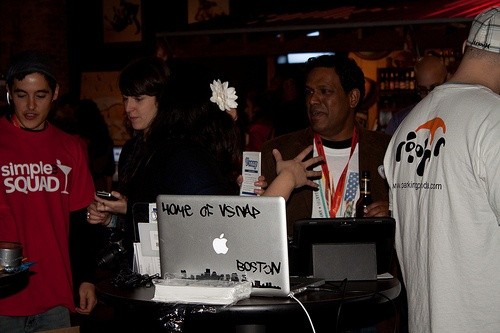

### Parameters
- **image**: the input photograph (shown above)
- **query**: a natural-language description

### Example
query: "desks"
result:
[95,272,401,325]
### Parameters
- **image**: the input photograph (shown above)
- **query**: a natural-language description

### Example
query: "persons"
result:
[86,56,191,274]
[383,6,500,332]
[259,54,392,333]
[0,64,98,333]
[176,93,324,208]
[26,46,115,195]
[384,56,451,136]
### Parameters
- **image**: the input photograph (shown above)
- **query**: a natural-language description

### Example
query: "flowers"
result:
[209,79,238,111]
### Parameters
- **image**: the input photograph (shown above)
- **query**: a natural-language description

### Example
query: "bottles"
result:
[440,49,460,65]
[356,172,374,217]
[380,67,416,90]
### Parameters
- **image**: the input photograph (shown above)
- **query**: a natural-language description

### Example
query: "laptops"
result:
[156,195,325,297]
[292,217,397,277]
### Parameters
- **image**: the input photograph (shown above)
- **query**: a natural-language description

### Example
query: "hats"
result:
[466,6,500,53]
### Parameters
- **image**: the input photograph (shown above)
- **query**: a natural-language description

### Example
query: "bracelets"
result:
[103,214,113,225]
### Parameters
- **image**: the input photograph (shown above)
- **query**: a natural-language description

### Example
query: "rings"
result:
[86,212,90,219]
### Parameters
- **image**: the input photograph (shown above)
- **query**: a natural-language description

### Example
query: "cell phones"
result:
[96,191,116,201]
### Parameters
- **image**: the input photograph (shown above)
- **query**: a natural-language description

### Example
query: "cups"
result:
[0,242,23,268]
